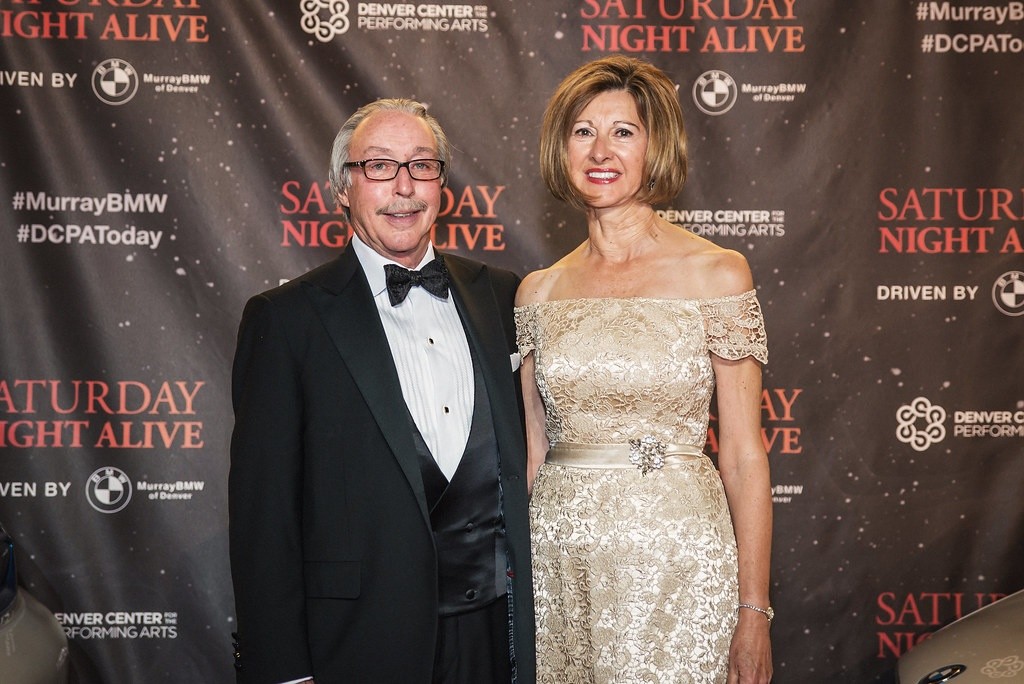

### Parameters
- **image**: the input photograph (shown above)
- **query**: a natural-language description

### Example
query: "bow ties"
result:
[384,254,449,307]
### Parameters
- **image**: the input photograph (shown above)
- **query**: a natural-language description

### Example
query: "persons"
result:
[514,55,775,684]
[223,98,535,684]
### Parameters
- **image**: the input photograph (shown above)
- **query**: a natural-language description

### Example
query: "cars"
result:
[895,590,1024,684]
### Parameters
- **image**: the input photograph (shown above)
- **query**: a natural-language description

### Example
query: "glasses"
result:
[344,158,444,182]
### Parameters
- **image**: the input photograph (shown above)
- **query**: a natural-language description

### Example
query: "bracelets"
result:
[736,604,774,623]
